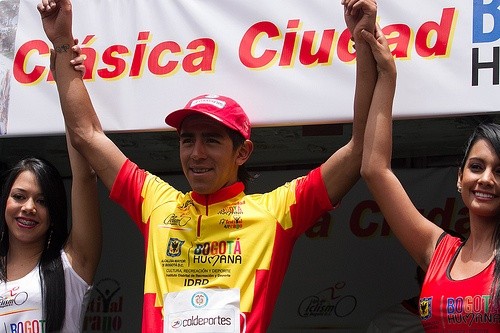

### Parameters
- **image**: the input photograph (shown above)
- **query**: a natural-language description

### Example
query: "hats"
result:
[165,94,251,139]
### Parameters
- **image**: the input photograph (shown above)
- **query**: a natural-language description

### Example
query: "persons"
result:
[1,38,102,333]
[347,19,500,333]
[36,0,380,333]
